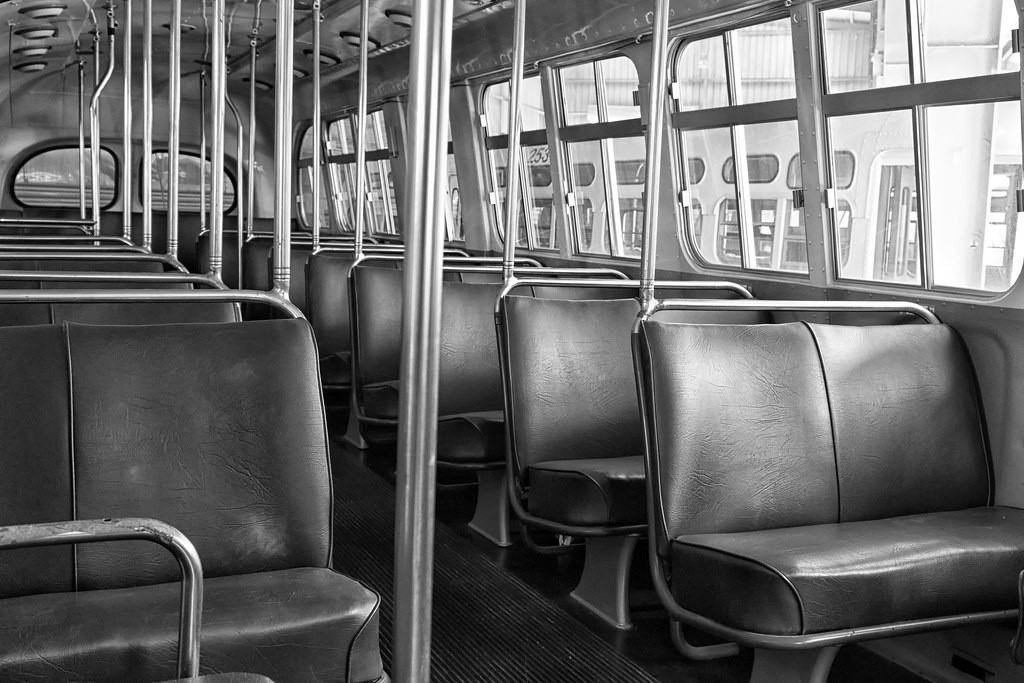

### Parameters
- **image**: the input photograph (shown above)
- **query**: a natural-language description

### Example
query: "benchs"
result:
[0,206,1023,683]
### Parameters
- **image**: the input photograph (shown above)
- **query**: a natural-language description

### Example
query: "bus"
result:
[303,0,1008,291]
